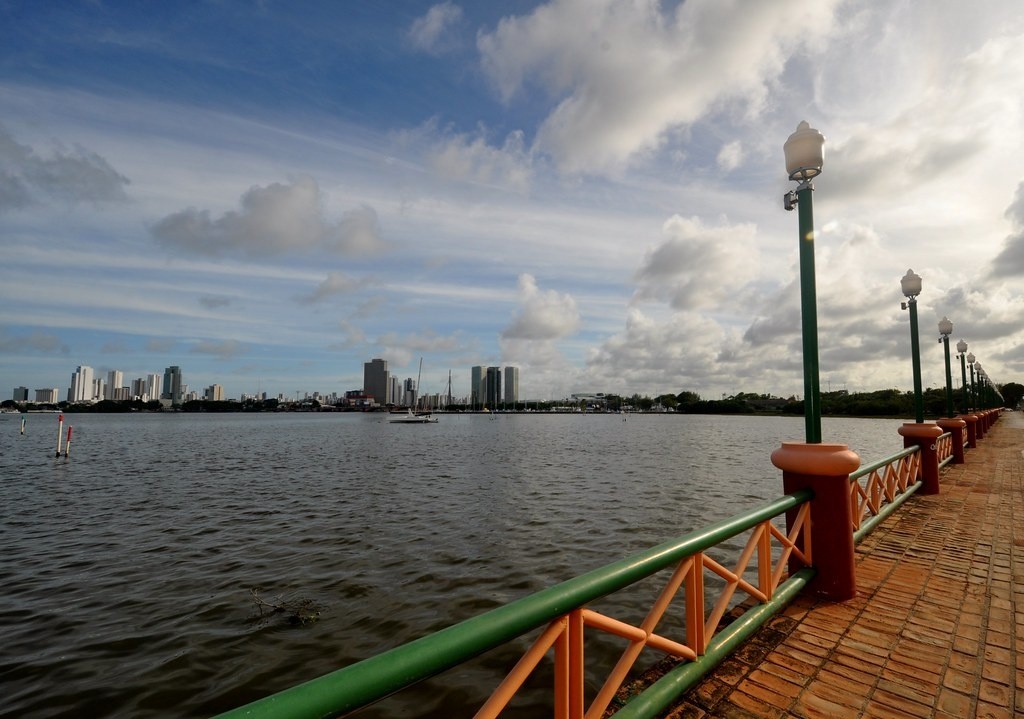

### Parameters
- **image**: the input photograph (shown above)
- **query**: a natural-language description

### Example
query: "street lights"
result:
[966,352,975,413]
[937,316,954,419]
[783,120,827,444]
[974,361,1005,410]
[956,338,969,415]
[900,268,923,423]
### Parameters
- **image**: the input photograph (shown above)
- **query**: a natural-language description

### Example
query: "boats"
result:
[385,408,438,424]
